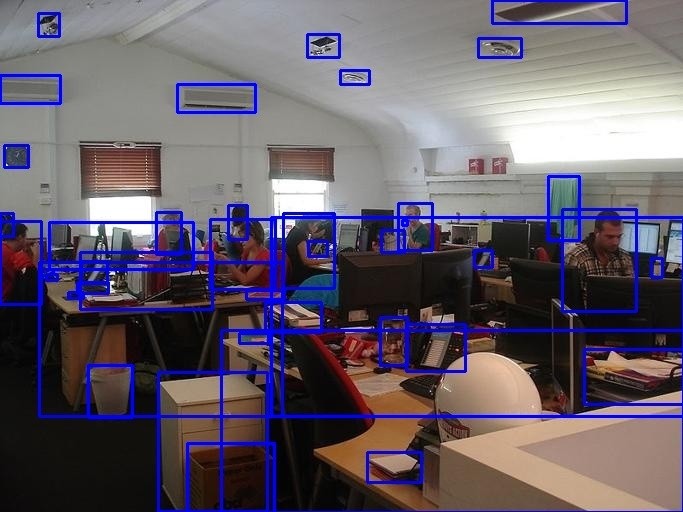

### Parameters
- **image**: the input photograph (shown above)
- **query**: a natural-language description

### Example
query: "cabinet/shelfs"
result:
[651,258,662,278]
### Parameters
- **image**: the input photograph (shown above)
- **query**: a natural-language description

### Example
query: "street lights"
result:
[382,230,404,253]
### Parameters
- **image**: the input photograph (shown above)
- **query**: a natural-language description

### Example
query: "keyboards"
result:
[77,283,109,294]
[40,269,53,276]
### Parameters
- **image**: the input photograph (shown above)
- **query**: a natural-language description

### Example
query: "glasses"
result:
[479,38,521,57]
[341,71,370,84]
[38,14,60,36]
[307,34,339,57]
[493,0,626,24]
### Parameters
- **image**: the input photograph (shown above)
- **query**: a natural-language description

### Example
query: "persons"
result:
[283,213,334,289]
[211,220,276,289]
[562,209,637,312]
[156,212,182,254]
[0,221,42,304]
[0,213,14,237]
[228,205,248,240]
[399,204,432,250]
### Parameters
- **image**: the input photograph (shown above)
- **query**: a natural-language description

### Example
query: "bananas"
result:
[77,283,109,294]
[40,269,53,276]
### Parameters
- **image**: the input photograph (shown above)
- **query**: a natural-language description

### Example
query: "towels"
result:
[187,443,275,512]
[88,366,133,417]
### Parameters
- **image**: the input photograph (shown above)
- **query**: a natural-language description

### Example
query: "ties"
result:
[50,222,194,269]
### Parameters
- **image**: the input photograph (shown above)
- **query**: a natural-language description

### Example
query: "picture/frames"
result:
[39,262,282,416]
[221,331,683,512]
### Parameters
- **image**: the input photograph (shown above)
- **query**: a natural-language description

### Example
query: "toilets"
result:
[82,261,108,283]
[407,324,465,372]
[475,250,492,268]
[309,242,327,257]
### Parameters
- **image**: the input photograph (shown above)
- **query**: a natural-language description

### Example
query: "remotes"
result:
[46,275,58,281]
[68,293,84,298]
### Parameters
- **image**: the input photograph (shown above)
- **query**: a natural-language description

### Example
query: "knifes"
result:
[0,76,61,103]
[177,85,256,112]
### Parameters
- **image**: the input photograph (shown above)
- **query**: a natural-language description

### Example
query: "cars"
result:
[380,317,407,366]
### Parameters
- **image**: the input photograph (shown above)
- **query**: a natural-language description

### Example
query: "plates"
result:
[159,373,267,512]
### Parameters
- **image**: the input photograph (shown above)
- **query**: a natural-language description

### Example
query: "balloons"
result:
[5,146,29,168]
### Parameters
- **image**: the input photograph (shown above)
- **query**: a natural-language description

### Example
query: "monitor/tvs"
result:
[585,350,683,404]
[491,222,531,260]
[585,275,682,358]
[240,334,268,344]
[527,220,562,262]
[50,222,194,269]
[368,453,422,483]
[618,222,661,274]
[247,291,285,299]
[551,299,587,413]
[80,253,212,309]
[266,302,322,332]
[508,255,584,318]
[360,208,394,236]
[421,248,473,324]
[337,249,422,328]
[295,217,334,244]
[469,300,555,386]
[451,224,478,248]
[664,220,683,274]
